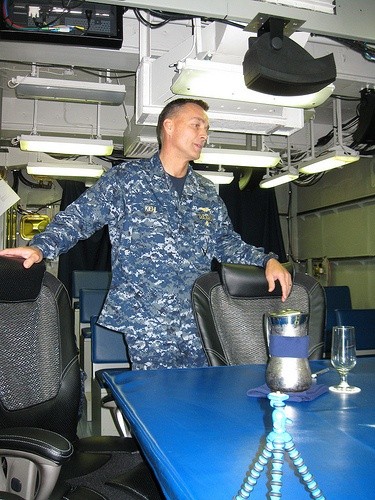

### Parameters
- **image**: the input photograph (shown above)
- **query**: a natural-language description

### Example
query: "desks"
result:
[104,357,375,500]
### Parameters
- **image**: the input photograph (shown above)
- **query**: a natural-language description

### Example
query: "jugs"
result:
[263,308,313,393]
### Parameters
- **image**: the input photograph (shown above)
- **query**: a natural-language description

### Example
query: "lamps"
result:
[259,136,299,188]
[194,130,281,167]
[170,17,335,108]
[8,62,126,106]
[11,98,114,156]
[26,152,104,179]
[298,96,360,174]
[194,164,235,184]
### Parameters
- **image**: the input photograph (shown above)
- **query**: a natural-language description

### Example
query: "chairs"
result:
[192,262,326,367]
[321,285,375,358]
[1,256,166,500]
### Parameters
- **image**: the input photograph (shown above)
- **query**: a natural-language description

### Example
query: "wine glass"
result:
[328,325,361,395]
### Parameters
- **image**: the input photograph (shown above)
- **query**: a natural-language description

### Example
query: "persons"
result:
[0,98,293,372]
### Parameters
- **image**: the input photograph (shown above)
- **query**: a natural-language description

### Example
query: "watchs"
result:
[268,252,279,260]
